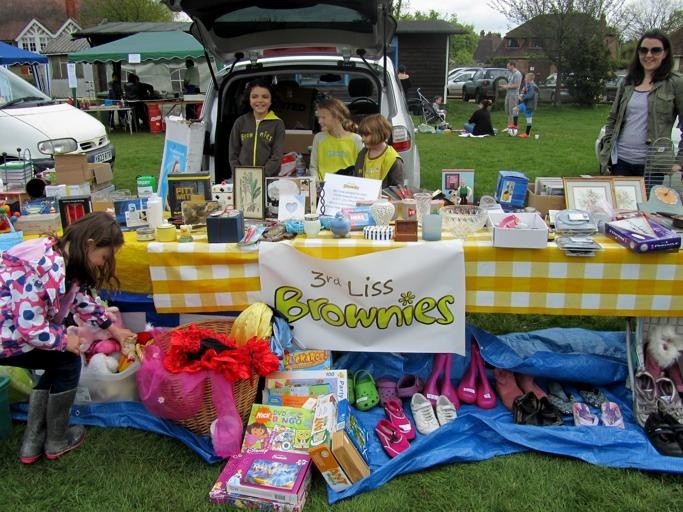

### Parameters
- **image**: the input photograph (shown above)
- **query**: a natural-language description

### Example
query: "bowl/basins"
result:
[440,204,486,236]
[23,205,42,215]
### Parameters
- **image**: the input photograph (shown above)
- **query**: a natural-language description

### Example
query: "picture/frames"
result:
[229,163,265,222]
[561,174,620,216]
[593,172,647,213]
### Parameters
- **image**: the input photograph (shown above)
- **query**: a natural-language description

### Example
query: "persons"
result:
[107,71,118,124]
[308,96,366,181]
[17,178,54,215]
[593,29,682,193]
[500,60,523,134]
[333,111,405,193]
[397,64,411,101]
[227,79,285,177]
[430,94,447,124]
[457,177,472,205]
[123,73,154,129]
[506,72,538,138]
[0,209,128,466]
[183,59,200,119]
[462,99,495,136]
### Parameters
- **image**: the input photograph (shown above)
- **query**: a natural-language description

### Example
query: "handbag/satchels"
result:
[592,123,611,164]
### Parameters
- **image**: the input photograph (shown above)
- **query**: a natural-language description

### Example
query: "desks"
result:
[0,204,682,410]
[181,101,206,118]
[85,107,132,135]
[127,98,186,132]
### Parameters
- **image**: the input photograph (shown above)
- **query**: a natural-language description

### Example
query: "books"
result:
[533,175,563,195]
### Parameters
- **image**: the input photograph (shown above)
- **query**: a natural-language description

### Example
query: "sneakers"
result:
[508,122,518,129]
[409,393,458,434]
[632,373,683,428]
[376,401,416,458]
[502,126,509,132]
[520,133,530,137]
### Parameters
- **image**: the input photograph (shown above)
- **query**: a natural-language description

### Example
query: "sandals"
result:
[511,393,567,428]
[343,371,426,412]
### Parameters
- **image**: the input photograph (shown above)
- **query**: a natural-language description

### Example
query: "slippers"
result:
[548,379,625,430]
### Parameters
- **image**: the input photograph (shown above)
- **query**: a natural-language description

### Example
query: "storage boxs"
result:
[492,169,530,211]
[203,212,249,244]
[113,193,150,228]
[603,214,682,255]
[166,169,213,217]
[280,131,314,159]
[264,176,318,216]
[519,181,568,213]
[484,209,550,252]
[0,149,120,244]
[211,340,381,510]
[67,349,142,409]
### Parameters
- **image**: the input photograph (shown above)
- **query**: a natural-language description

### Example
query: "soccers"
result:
[506,127,518,138]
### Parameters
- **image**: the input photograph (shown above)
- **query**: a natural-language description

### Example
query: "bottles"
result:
[146,192,163,228]
[177,222,194,242]
[155,219,176,241]
[303,214,321,237]
[124,203,139,228]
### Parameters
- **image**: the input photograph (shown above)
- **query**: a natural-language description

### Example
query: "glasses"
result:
[638,44,666,56]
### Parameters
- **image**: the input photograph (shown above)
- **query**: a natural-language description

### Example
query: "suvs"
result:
[163,2,419,199]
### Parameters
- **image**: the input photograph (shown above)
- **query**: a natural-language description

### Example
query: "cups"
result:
[534,134,539,140]
[421,214,441,240]
[65,203,84,226]
[382,193,441,227]
[96,188,132,201]
[479,195,497,208]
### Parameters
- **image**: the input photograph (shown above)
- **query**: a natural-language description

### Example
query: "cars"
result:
[446,67,626,104]
[0,64,115,176]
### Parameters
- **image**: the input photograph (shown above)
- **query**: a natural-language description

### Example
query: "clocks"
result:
[646,182,681,208]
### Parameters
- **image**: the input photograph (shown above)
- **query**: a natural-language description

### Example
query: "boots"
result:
[21,387,46,464]
[425,350,461,407]
[497,367,547,411]
[644,326,683,393]
[45,390,86,460]
[455,335,497,409]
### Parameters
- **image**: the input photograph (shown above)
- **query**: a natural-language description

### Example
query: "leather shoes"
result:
[642,413,683,457]
